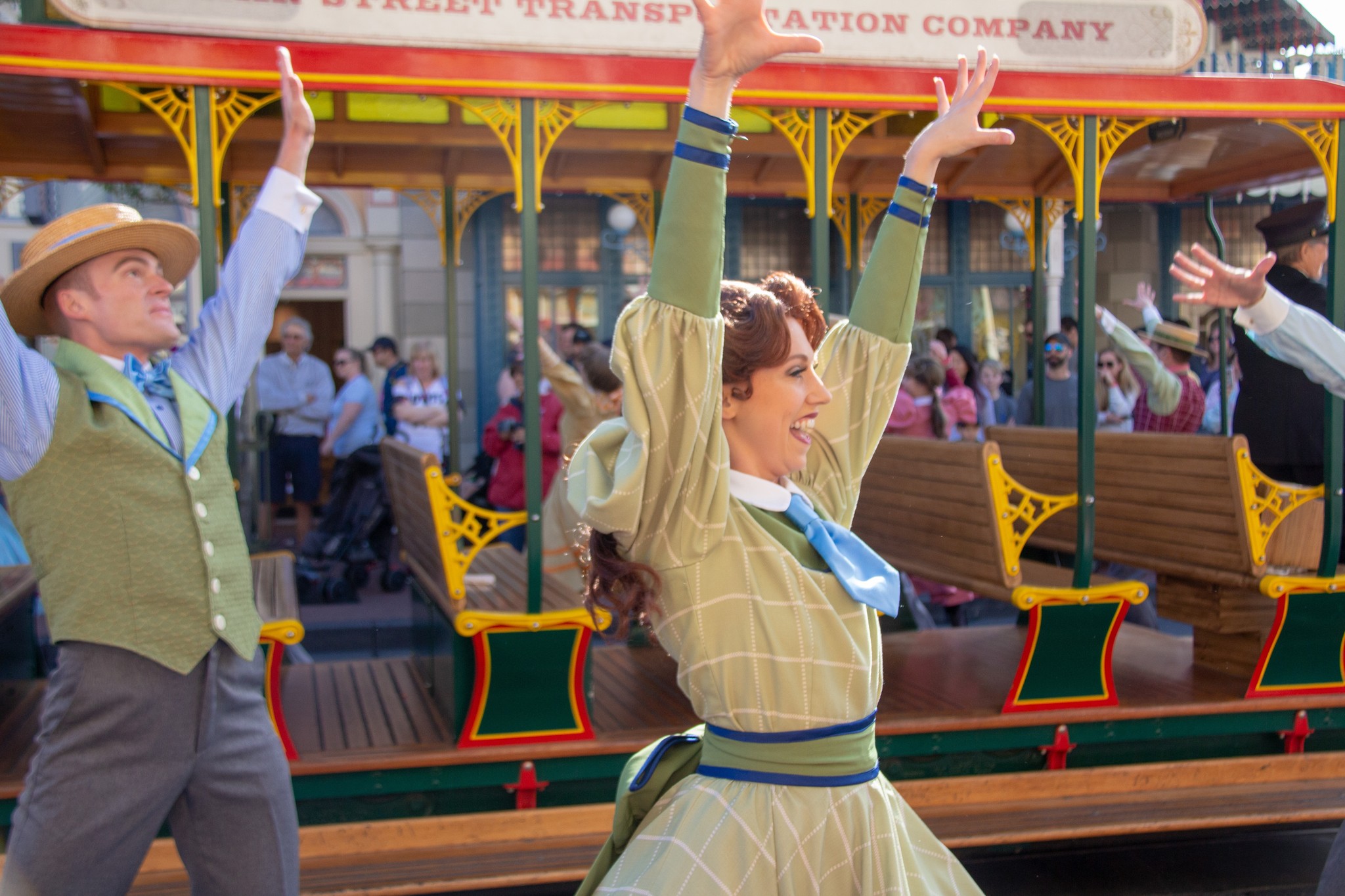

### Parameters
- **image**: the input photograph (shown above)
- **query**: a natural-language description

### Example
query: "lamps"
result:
[601,201,640,253]
[999,206,1037,250]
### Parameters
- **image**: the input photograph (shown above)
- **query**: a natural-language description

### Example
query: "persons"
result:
[1230,193,1334,487]
[0,43,326,895]
[1167,243,1345,402]
[244,315,625,589]
[559,0,1016,896]
[878,279,1245,448]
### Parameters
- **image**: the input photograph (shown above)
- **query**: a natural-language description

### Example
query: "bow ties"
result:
[123,352,175,401]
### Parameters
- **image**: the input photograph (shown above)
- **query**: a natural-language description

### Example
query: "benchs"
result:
[375,437,613,747]
[980,422,1345,698]
[848,430,1147,712]
[243,547,308,765]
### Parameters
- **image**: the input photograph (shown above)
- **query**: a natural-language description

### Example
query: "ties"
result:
[785,493,901,618]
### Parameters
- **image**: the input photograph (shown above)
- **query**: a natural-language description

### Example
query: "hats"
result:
[366,338,397,352]
[1046,331,1071,346]
[1254,195,1331,247]
[1140,323,1210,359]
[1,202,198,337]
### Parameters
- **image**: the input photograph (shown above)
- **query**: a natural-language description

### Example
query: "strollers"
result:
[287,445,422,599]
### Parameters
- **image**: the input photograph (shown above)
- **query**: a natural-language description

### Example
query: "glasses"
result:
[330,359,358,365]
[1045,344,1067,352]
[1209,332,1221,346]
[1098,360,1119,367]
[283,332,305,340]
